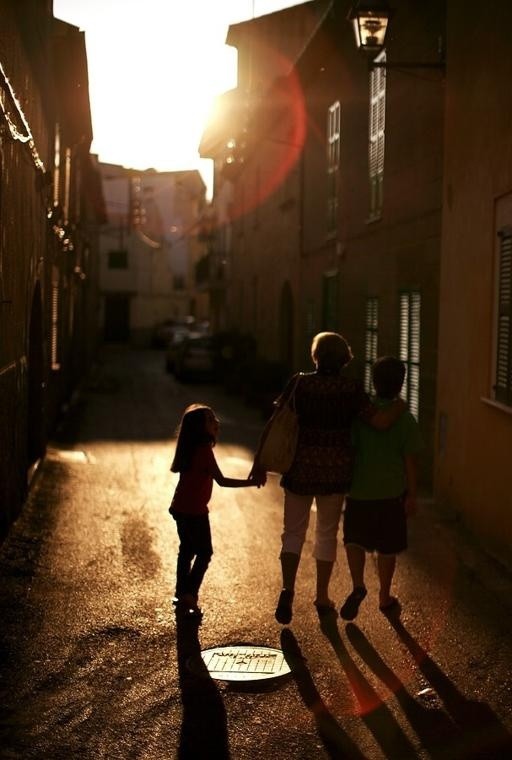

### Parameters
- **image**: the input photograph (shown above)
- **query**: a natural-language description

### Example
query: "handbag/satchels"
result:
[259,407,300,473]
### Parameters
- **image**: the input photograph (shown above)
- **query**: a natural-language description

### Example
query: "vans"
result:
[166,330,215,384]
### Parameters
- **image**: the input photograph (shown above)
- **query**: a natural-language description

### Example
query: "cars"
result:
[155,315,210,346]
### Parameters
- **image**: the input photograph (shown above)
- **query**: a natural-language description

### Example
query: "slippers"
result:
[172,600,203,617]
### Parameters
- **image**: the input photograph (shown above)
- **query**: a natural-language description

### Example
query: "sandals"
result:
[339,587,367,620]
[313,599,335,610]
[275,588,293,624]
[379,595,399,609]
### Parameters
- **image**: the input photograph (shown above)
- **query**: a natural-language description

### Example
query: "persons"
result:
[341,359,418,620]
[255,332,407,625]
[169,406,265,605]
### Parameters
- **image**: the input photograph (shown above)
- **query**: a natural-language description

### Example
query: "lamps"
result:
[346,3,446,68]
